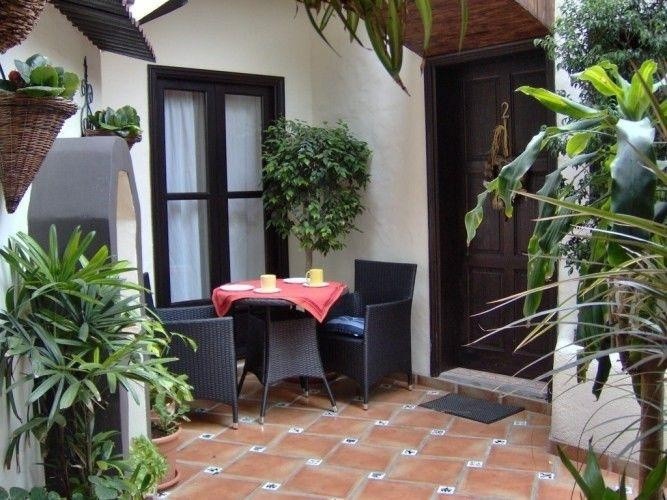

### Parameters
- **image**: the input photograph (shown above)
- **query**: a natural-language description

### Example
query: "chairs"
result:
[144,272,238,429]
[305,259,417,410]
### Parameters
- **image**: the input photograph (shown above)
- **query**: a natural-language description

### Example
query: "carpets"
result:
[419,392,527,423]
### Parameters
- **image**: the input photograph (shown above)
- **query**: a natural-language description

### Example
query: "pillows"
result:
[324,315,365,337]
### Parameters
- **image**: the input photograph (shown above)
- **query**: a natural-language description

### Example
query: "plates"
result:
[219,277,330,294]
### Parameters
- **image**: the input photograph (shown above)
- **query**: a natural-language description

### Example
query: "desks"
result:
[212,278,350,424]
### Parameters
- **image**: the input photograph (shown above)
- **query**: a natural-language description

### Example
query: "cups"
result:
[306,269,324,285]
[260,274,277,290]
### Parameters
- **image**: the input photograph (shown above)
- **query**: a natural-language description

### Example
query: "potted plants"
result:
[142,304,198,492]
